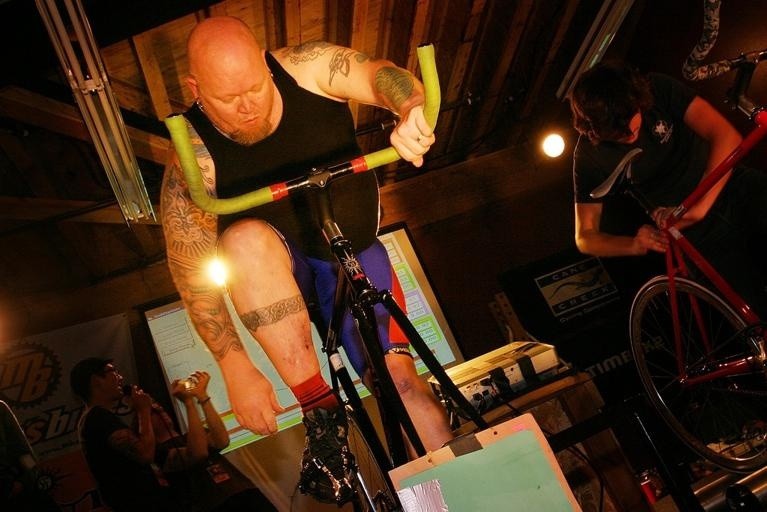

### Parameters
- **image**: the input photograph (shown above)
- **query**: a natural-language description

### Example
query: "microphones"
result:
[121,383,157,417]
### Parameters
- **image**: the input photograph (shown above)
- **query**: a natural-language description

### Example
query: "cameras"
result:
[177,377,198,392]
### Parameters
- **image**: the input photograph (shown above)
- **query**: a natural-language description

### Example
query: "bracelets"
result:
[196,396,211,406]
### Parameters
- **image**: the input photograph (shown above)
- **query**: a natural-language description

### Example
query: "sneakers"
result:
[298,401,357,505]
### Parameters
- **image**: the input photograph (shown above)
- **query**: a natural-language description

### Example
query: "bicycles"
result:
[579,1,765,483]
[161,37,499,512]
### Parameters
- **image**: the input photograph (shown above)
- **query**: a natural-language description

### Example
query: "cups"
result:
[641,480,658,508]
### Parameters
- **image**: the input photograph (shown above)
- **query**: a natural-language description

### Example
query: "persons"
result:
[67,354,176,512]
[130,370,278,512]
[565,59,767,380]
[158,12,454,506]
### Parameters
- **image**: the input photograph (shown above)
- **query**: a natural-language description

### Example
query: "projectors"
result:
[427,339,559,416]
